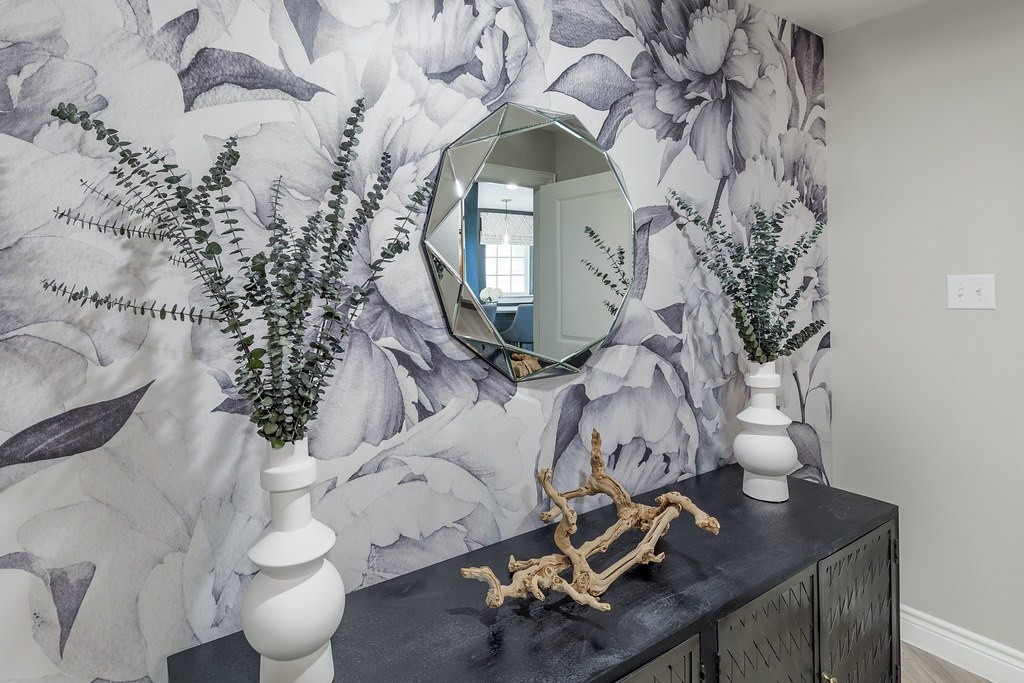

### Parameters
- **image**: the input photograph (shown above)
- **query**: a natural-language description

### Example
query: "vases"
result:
[486,301,498,310]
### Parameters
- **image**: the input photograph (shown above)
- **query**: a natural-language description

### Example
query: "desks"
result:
[495,306,520,314]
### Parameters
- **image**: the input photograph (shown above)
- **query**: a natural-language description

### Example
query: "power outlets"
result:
[944,273,996,309]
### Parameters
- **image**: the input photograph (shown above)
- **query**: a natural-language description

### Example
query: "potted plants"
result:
[42,94,438,682]
[668,183,826,501]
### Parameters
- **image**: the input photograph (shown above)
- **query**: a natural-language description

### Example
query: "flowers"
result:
[479,287,502,301]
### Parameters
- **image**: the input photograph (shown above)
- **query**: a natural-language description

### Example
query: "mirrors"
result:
[421,102,636,384]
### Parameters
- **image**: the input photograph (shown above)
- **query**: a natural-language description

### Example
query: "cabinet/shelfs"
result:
[718,517,898,683]
[614,632,702,682]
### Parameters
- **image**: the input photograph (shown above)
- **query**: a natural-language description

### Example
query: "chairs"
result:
[482,304,497,323]
[500,304,534,347]
